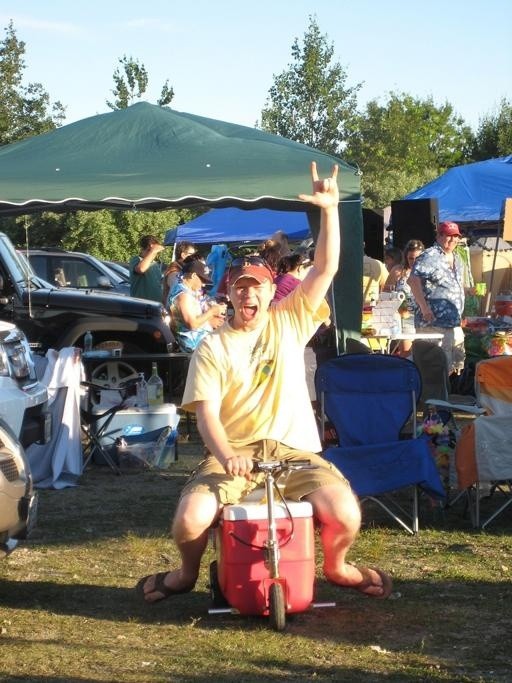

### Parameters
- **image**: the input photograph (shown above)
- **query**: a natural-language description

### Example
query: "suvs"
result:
[0,232,172,424]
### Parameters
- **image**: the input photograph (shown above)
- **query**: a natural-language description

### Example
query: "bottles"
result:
[83,330,93,352]
[147,360,164,410]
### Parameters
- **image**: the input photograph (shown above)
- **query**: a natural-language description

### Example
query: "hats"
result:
[183,260,214,284]
[437,222,462,237]
[226,257,275,284]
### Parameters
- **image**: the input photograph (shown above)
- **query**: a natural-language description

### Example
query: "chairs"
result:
[315,337,512,535]
[78,380,132,476]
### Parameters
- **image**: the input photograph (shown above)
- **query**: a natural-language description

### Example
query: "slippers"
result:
[342,560,393,601]
[135,572,194,605]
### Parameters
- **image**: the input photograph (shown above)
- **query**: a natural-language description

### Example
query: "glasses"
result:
[227,256,274,277]
[409,243,424,250]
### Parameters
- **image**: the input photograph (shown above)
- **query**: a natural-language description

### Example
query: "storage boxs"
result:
[91,403,177,466]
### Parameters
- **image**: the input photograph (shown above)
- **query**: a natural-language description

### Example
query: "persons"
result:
[129,234,331,413]
[359,221,479,395]
[136,159,395,604]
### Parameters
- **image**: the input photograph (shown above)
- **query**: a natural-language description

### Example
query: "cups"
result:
[475,282,487,297]
